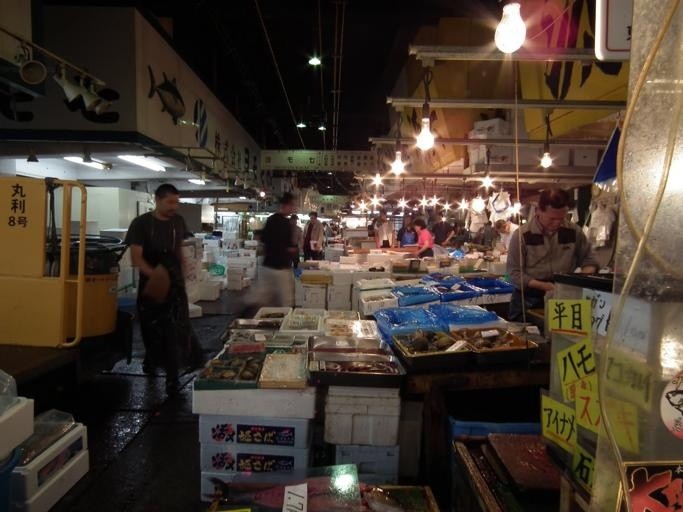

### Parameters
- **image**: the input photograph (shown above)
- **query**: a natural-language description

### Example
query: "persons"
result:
[305,211,323,261]
[396,216,417,248]
[374,209,393,249]
[488,222,511,254]
[286,216,304,273]
[433,213,452,245]
[445,220,470,251]
[412,219,432,257]
[507,190,600,323]
[259,192,298,310]
[484,222,499,251]
[126,184,205,399]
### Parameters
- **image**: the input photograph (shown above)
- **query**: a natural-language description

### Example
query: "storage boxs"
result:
[280,314,320,333]
[309,352,405,390]
[0,396,35,462]
[374,308,446,339]
[200,441,308,481]
[433,284,484,309]
[468,280,513,305]
[351,271,390,279]
[428,305,505,333]
[13,424,90,512]
[450,327,540,369]
[202,472,236,504]
[228,279,243,291]
[301,271,333,286]
[221,319,283,342]
[181,286,200,304]
[175,229,258,284]
[322,386,401,448]
[254,307,292,319]
[200,281,220,300]
[318,316,380,342]
[333,271,350,286]
[356,292,399,315]
[355,279,394,291]
[305,252,489,272]
[418,275,466,288]
[197,412,309,449]
[326,285,351,309]
[391,335,471,374]
[301,284,327,308]
[190,343,316,419]
[394,286,440,307]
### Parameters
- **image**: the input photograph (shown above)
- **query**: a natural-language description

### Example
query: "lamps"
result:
[416,60,436,151]
[541,106,554,169]
[483,171,491,189]
[491,1,526,54]
[376,149,381,186]
[390,112,406,176]
